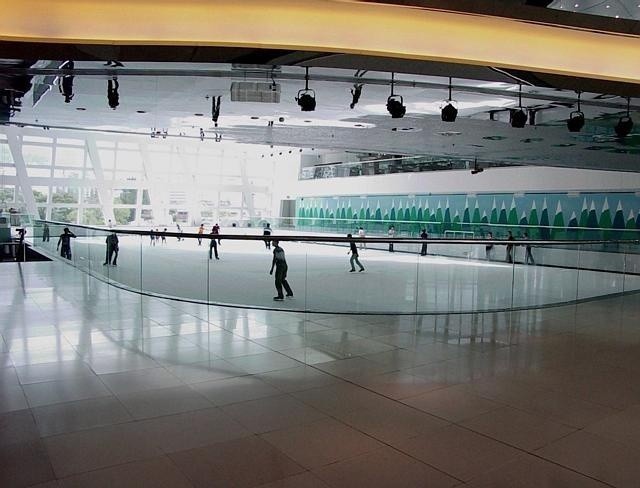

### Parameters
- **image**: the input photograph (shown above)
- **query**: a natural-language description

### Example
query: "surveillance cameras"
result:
[471,169,483,174]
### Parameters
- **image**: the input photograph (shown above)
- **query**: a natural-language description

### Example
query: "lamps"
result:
[566,86,585,132]
[295,80,317,112]
[385,84,406,119]
[614,94,634,138]
[510,82,528,128]
[440,85,459,122]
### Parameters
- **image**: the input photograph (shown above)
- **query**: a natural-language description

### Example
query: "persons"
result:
[485,231,493,262]
[42,223,49,242]
[359,227,366,250]
[150,223,221,260]
[523,232,535,266]
[505,230,514,264]
[270,241,294,300]
[57,228,76,260]
[420,229,428,256]
[388,225,395,252]
[103,234,119,266]
[346,233,365,273]
[263,223,272,249]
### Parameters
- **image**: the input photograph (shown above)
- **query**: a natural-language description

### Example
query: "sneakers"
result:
[349,269,365,272]
[273,292,293,299]
